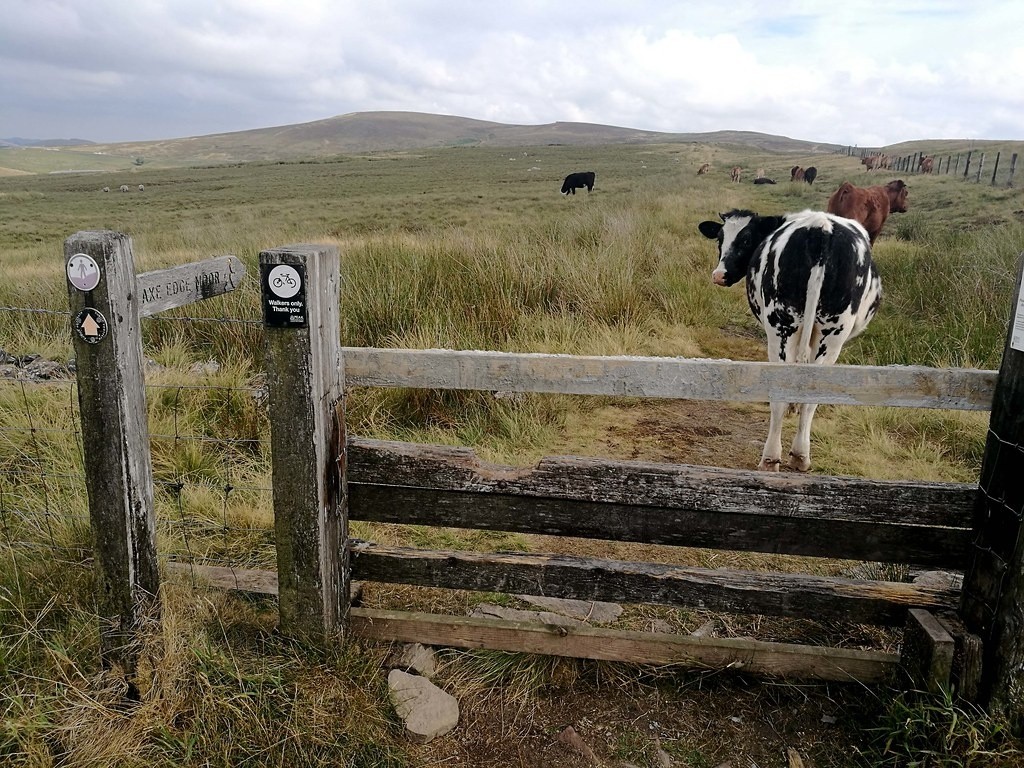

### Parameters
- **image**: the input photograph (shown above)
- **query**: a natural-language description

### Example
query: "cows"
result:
[918,156,934,174]
[696,162,710,175]
[861,155,882,172]
[789,165,804,181]
[754,177,777,185]
[560,171,596,195]
[697,208,883,472]
[826,178,909,246]
[805,166,818,186]
[731,165,742,183]
[755,167,765,177]
